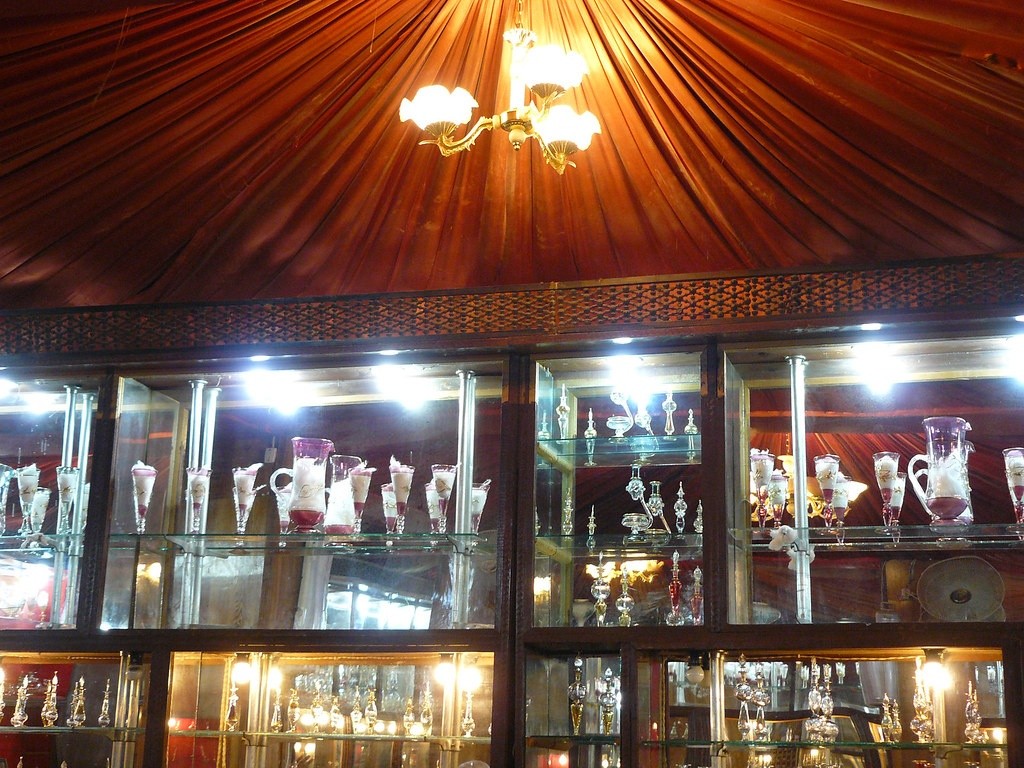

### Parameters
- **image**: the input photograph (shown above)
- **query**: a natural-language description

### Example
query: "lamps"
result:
[398,0,602,176]
[586,548,665,587]
[749,433,868,522]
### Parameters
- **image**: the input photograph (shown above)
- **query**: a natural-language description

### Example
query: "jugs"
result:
[913,441,976,532]
[270,437,335,538]
[325,455,367,545]
[908,417,972,531]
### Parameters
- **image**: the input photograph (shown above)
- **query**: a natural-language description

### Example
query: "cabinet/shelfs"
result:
[0,352,522,768]
[511,328,1024,768]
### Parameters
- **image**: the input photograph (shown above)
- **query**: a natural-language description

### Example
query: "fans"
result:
[751,601,781,624]
[925,600,1006,622]
[916,555,1006,622]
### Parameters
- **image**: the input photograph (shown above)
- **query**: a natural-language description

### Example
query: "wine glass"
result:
[885,472,908,542]
[471,484,487,546]
[872,452,901,533]
[348,468,377,541]
[432,464,457,534]
[381,483,397,546]
[1003,447,1024,533]
[769,475,790,530]
[828,476,852,542]
[232,468,259,538]
[0,463,92,537]
[128,468,158,541]
[389,464,414,538]
[229,487,257,555]
[274,488,292,548]
[185,468,214,539]
[423,483,445,552]
[813,454,841,535]
[749,454,777,529]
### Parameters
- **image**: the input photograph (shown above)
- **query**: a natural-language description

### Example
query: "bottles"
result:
[684,409,698,462]
